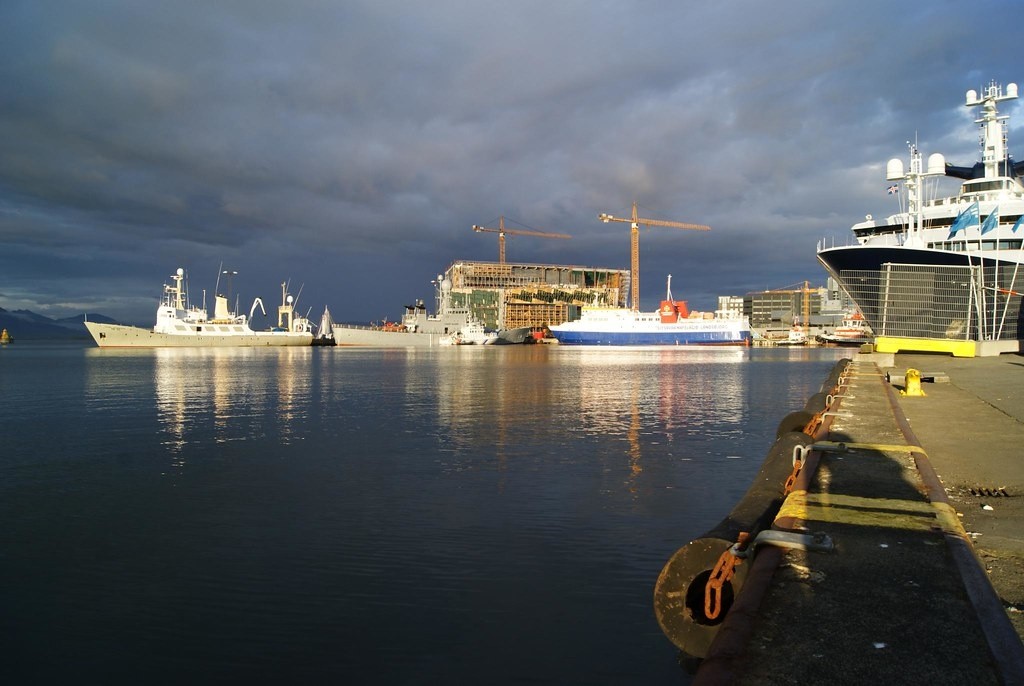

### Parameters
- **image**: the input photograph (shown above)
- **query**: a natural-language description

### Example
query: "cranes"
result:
[746,280,823,326]
[598,201,712,310]
[472,215,572,264]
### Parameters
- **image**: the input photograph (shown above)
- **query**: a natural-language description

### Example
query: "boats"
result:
[815,308,876,346]
[812,81,1024,357]
[546,293,754,347]
[83,259,319,350]
[437,310,500,346]
[329,273,533,347]
[775,313,809,346]
[308,305,336,346]
[0,327,16,345]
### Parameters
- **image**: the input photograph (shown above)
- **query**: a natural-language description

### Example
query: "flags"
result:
[977,205,998,235]
[947,210,961,239]
[886,184,898,194]
[1011,214,1024,233]
[948,202,979,232]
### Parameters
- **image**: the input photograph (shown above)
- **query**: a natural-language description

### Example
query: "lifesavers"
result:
[815,336,825,343]
